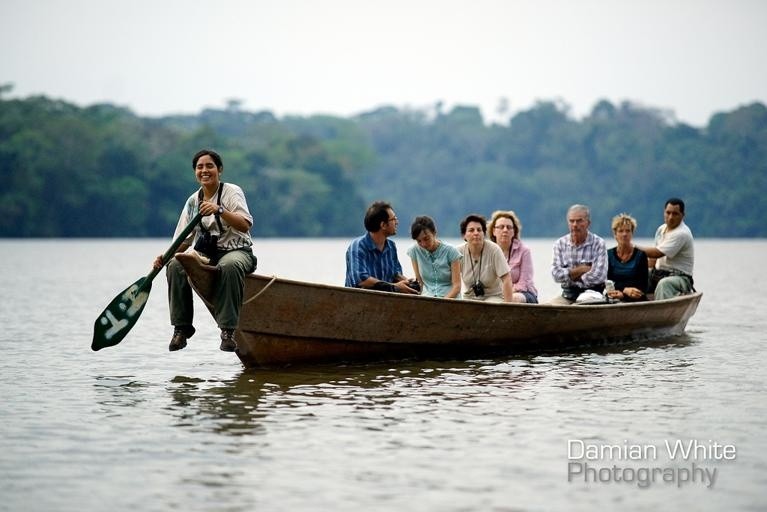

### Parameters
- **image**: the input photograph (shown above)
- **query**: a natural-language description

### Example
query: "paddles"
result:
[91,202,210,352]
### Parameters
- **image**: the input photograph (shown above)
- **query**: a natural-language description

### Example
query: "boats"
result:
[175,252,703,368]
[213,356,451,433]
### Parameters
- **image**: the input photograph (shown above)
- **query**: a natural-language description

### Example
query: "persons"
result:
[486,210,538,304]
[344,201,421,294]
[606,212,649,304]
[634,198,696,301]
[153,149,257,352]
[407,215,464,299]
[552,204,609,306]
[459,214,512,304]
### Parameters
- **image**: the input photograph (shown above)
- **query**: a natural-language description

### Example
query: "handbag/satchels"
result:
[562,262,605,301]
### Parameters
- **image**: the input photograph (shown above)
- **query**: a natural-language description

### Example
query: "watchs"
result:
[215,207,224,216]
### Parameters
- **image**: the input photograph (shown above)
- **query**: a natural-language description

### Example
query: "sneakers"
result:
[220,328,237,352]
[169,326,195,351]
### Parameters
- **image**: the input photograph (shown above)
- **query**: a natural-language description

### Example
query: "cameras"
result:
[407,281,420,292]
[473,280,484,295]
[194,233,218,257]
